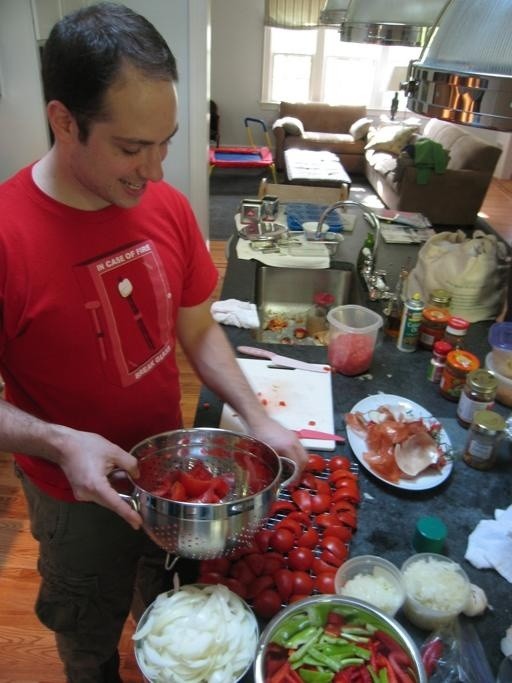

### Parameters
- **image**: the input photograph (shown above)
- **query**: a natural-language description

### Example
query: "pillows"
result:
[364,123,418,156]
[349,115,373,138]
[281,115,306,136]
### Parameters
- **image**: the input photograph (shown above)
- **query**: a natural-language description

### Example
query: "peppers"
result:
[421,637,443,677]
[266,604,416,683]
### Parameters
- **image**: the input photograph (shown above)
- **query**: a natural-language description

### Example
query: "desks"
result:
[160,206,512,682]
[207,146,276,185]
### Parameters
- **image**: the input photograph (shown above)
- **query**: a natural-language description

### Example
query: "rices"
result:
[404,556,466,632]
[342,573,401,618]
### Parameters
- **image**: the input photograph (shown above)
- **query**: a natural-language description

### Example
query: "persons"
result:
[0,0,313,683]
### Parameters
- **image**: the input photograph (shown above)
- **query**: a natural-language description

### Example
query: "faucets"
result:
[316,199,382,280]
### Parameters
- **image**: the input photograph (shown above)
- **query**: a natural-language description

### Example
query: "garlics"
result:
[462,584,487,618]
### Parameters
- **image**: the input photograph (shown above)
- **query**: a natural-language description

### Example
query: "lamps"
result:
[388,64,411,123]
[398,0,512,133]
[336,0,448,49]
[317,0,348,26]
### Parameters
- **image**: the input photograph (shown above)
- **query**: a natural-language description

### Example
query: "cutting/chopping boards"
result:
[217,356,338,452]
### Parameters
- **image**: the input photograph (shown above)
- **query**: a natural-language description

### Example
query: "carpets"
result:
[208,164,512,251]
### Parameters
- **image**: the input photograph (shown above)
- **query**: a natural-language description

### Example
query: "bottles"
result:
[356,230,377,275]
[410,515,448,559]
[383,269,410,342]
[395,289,507,475]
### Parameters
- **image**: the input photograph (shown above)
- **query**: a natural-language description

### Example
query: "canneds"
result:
[417,290,506,470]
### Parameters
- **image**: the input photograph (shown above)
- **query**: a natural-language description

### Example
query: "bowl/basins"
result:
[131,585,258,680]
[334,554,406,616]
[395,553,473,632]
[301,221,330,237]
[251,596,428,682]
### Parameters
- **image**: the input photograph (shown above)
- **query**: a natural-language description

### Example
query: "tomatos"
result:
[149,437,361,617]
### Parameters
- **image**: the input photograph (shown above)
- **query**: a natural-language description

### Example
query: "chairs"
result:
[257,176,350,215]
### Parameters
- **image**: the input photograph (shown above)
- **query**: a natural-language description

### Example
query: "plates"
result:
[345,393,455,492]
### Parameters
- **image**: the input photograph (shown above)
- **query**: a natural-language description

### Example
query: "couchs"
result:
[364,115,505,217]
[270,98,377,176]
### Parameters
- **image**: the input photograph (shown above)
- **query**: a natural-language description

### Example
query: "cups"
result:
[326,303,385,376]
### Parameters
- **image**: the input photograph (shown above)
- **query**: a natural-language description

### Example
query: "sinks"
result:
[252,261,368,347]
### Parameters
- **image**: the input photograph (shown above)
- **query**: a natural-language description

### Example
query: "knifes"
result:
[236,345,330,373]
[288,428,346,444]
